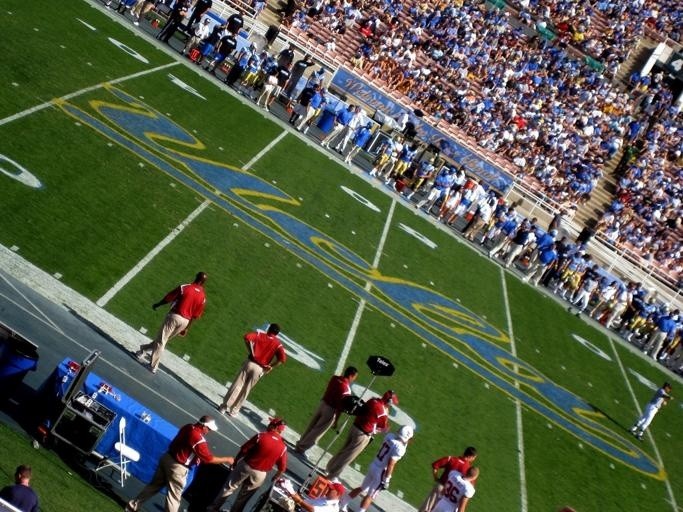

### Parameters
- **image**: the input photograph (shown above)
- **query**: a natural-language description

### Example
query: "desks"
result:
[0,321,308,512]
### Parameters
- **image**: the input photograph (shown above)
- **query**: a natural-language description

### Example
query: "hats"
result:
[327,483,345,495]
[198,419,218,432]
[384,392,399,404]
[269,417,285,430]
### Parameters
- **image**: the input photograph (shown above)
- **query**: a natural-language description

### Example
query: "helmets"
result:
[397,425,413,443]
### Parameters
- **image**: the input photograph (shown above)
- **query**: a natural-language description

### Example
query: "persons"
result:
[105,1,683,374]
[123,411,233,511]
[628,381,674,442]
[0,462,40,511]
[217,319,287,416]
[294,365,482,511]
[209,417,288,511]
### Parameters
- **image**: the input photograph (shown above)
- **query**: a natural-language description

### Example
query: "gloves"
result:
[280,478,296,496]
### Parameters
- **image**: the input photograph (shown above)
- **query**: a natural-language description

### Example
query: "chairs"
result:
[113,0,682,348]
